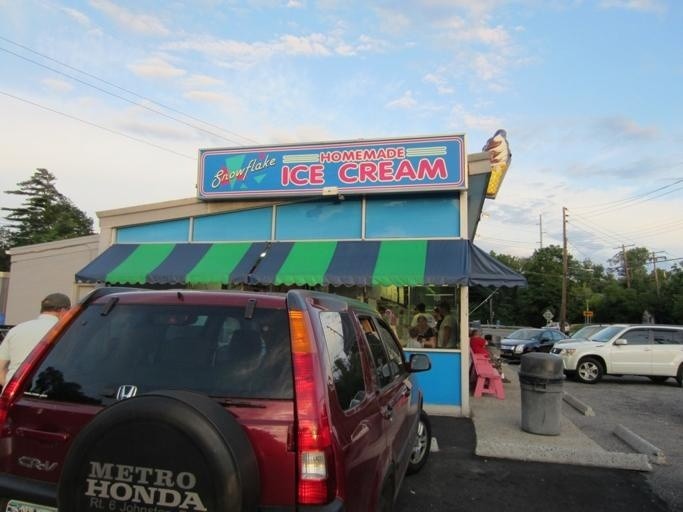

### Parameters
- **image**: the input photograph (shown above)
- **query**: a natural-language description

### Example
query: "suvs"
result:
[0,286,433,512]
[549,323,683,388]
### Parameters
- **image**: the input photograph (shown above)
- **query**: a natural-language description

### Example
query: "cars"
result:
[500,321,611,365]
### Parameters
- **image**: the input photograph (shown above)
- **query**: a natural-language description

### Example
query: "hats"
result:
[41,293,71,311]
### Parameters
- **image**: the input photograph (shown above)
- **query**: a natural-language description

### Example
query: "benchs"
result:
[468,346,505,398]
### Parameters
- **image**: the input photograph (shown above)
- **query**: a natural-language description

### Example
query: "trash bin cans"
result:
[518,352,566,436]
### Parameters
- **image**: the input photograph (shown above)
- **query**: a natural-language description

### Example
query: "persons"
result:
[0,293,74,397]
[470,328,511,383]
[483,334,496,348]
[383,298,457,349]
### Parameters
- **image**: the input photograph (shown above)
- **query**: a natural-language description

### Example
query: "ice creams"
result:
[482,129,512,198]
